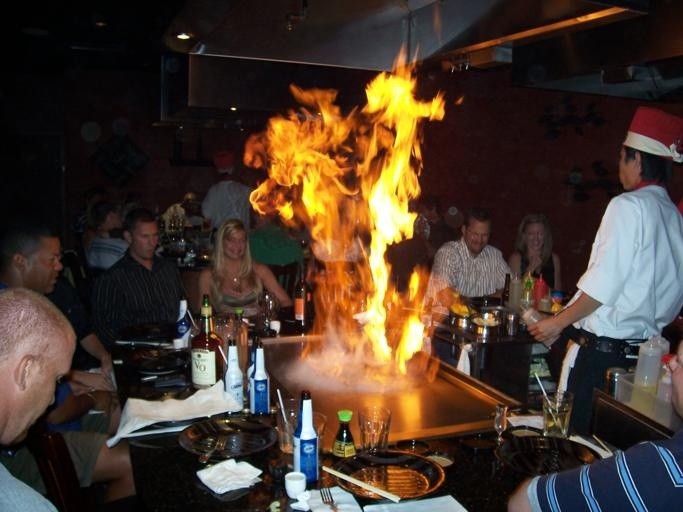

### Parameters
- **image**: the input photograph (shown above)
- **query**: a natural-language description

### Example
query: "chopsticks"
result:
[318,465,402,504]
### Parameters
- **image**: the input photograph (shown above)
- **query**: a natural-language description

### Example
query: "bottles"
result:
[502,274,511,307]
[232,308,248,371]
[293,390,320,490]
[249,341,270,414]
[522,279,533,310]
[509,273,521,311]
[524,271,533,287]
[506,313,519,337]
[550,292,563,312]
[333,410,356,462]
[534,274,547,310]
[173,296,191,353]
[605,369,627,395]
[224,339,244,418]
[294,262,313,333]
[631,337,662,413]
[202,294,211,308]
[651,334,670,358]
[520,304,561,347]
[656,355,676,404]
[248,336,260,408]
[191,307,224,394]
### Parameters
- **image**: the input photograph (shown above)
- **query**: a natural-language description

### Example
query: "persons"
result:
[85,199,128,268]
[424,206,514,312]
[79,207,188,373]
[197,219,293,320]
[509,211,561,293]
[510,338,682,512]
[2,220,136,502]
[0,286,77,510]
[204,143,251,229]
[527,106,682,400]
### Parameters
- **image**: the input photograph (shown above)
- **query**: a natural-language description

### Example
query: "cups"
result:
[283,469,307,499]
[273,405,298,455]
[291,410,326,452]
[263,298,281,332]
[358,405,393,454]
[214,313,234,355]
[542,390,574,439]
[493,404,508,443]
[258,290,277,328]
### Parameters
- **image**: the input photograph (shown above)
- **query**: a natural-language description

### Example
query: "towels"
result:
[106,379,242,448]
[197,459,263,495]
[363,495,468,511]
[290,487,360,512]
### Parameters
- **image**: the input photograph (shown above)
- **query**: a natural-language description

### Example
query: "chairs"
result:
[583,389,677,455]
[25,428,82,512]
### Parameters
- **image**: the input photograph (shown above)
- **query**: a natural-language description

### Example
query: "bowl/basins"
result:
[450,316,471,338]
[467,312,502,344]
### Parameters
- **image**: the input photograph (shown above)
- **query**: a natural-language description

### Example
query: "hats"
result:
[623,108,683,163]
[217,155,232,174]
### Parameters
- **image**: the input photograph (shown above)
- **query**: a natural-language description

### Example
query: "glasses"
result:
[465,227,490,239]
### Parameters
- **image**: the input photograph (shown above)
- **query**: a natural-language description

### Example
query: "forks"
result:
[198,434,228,464]
[317,484,338,512]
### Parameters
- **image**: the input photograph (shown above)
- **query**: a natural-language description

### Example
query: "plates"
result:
[421,448,457,469]
[135,354,188,376]
[506,424,544,438]
[178,414,277,460]
[493,436,605,477]
[392,437,431,458]
[334,450,446,502]
